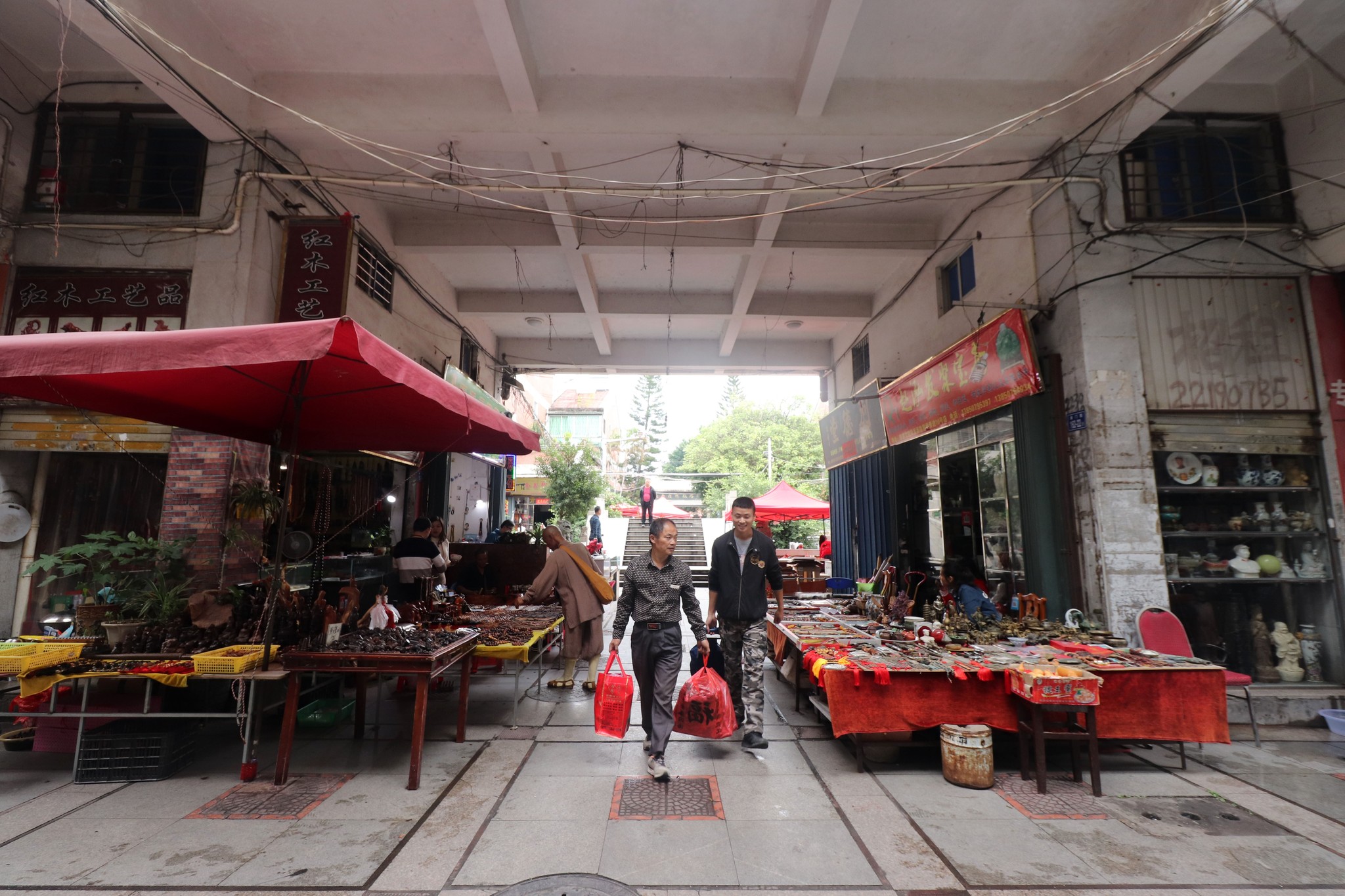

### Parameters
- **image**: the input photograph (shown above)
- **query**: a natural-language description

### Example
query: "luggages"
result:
[690,627,724,680]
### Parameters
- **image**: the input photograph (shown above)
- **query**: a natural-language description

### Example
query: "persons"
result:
[640,479,656,527]
[609,518,711,778]
[517,523,526,532]
[485,520,515,543]
[428,516,459,590]
[589,506,603,550]
[935,561,1001,621]
[704,499,784,748]
[514,524,605,692]
[389,515,447,600]
[819,535,832,560]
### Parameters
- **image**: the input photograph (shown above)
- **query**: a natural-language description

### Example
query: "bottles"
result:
[865,594,885,621]
[1253,502,1289,532]
[789,542,795,549]
[1233,453,1284,487]
[1298,624,1325,682]
[798,543,803,549]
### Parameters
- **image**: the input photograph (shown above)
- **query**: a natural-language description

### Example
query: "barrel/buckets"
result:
[914,622,932,638]
[856,581,874,593]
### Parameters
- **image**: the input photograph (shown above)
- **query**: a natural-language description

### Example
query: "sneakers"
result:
[647,752,670,780]
[643,734,652,750]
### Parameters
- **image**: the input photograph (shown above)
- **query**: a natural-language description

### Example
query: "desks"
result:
[764,595,1232,770]
[0,604,564,790]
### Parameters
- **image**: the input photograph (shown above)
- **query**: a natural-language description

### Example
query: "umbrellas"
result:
[0,316,542,670]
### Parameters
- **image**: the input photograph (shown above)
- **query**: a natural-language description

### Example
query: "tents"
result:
[608,496,691,518]
[724,480,830,534]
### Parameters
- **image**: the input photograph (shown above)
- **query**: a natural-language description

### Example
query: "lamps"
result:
[503,377,525,391]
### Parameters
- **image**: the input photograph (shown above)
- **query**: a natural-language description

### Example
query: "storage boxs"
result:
[0,642,86,673]
[73,731,196,784]
[0,642,37,652]
[32,692,160,754]
[191,644,280,674]
[261,555,393,593]
[1005,663,1104,706]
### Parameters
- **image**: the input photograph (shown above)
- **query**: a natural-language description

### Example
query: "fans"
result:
[281,532,313,560]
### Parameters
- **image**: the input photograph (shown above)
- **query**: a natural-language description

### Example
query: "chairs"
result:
[1017,593,1047,622]
[1137,606,1262,770]
[783,577,857,598]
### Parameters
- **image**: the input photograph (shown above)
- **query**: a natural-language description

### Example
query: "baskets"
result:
[0,641,87,674]
[190,644,280,674]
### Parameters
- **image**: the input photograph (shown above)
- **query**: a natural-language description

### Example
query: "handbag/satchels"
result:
[594,651,634,739]
[673,653,737,739]
[559,545,615,605]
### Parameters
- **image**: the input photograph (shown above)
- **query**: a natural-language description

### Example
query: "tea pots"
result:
[875,628,915,641]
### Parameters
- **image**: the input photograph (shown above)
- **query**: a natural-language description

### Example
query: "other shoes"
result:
[547,679,574,689]
[735,713,745,729]
[641,524,645,527]
[582,681,597,692]
[742,730,768,749]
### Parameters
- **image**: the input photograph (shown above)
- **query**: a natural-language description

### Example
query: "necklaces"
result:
[499,605,564,630]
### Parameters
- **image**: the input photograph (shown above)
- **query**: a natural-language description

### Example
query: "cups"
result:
[1201,466,1218,487]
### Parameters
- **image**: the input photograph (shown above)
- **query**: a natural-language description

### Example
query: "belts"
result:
[637,622,678,630]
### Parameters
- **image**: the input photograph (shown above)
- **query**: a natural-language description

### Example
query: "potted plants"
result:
[22,476,284,649]
[369,526,394,556]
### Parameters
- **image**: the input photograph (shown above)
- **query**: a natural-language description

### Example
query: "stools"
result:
[1014,694,1102,798]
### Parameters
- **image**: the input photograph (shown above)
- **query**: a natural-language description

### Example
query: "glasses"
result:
[506,526,513,531]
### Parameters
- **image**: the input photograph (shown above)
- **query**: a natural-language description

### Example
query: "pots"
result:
[36,604,76,637]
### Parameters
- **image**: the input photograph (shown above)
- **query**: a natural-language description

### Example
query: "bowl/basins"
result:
[0,637,23,647]
[450,554,462,562]
[1007,637,1029,647]
[904,616,925,630]
[949,636,967,646]
[0,727,37,751]
[1092,635,1128,647]
[1318,709,1345,737]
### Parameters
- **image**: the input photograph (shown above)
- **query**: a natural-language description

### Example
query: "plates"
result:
[1088,630,1114,637]
[874,635,919,644]
[1058,658,1082,664]
[1165,452,1202,485]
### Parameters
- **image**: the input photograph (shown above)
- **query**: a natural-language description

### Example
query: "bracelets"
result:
[477,628,534,646]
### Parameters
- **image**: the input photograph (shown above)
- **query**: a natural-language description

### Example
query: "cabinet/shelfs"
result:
[1155,448,1345,689]
